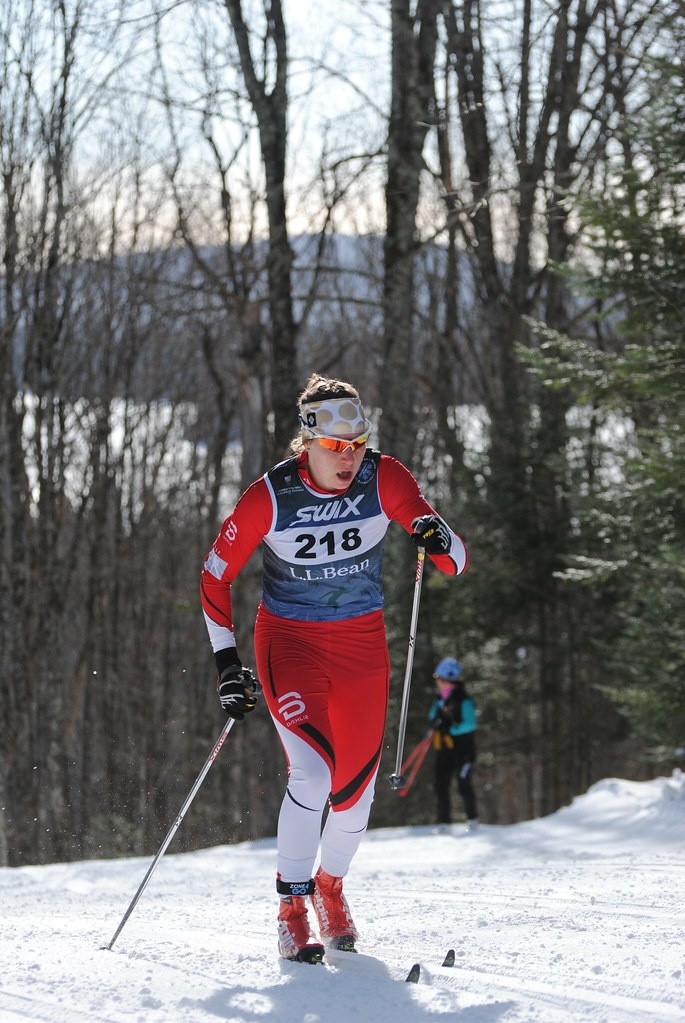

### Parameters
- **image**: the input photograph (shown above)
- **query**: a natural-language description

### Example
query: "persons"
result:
[428,657,478,830]
[200,373,468,963]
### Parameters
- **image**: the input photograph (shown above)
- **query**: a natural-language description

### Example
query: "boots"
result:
[276,871,326,963]
[309,864,358,949]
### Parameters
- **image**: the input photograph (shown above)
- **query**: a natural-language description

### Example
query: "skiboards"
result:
[201,931,458,985]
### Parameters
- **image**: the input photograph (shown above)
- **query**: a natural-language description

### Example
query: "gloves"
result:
[213,647,263,721]
[433,708,451,735]
[409,514,452,555]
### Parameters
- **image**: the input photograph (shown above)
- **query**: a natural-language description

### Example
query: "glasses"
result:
[304,418,373,455]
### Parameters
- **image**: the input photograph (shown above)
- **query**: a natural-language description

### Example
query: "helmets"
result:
[434,656,464,682]
